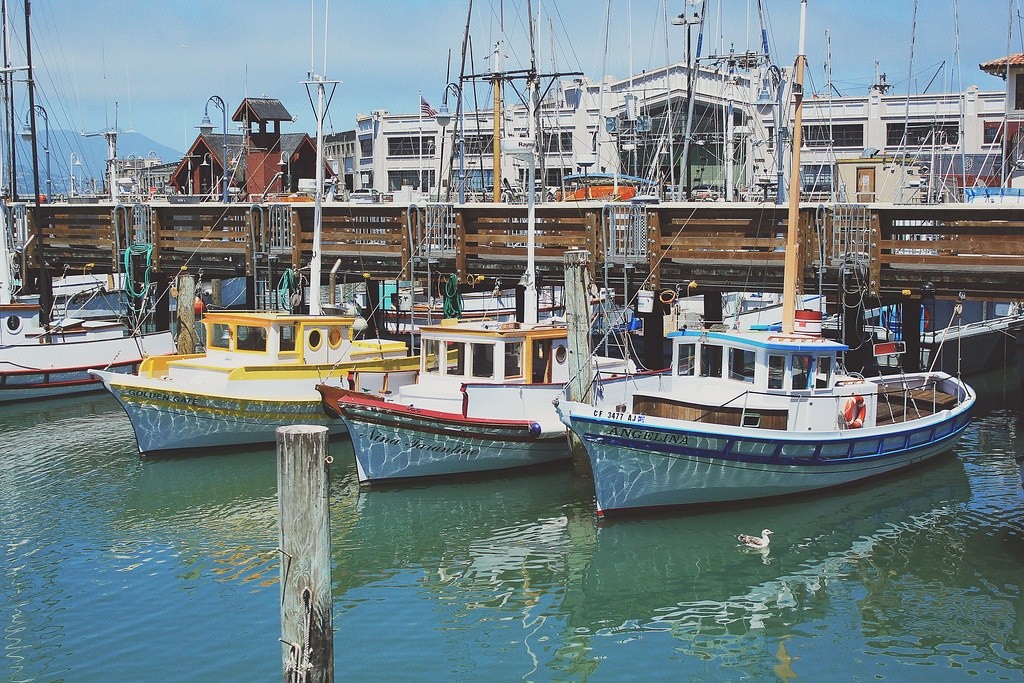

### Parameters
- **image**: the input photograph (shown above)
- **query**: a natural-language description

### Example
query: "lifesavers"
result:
[843,393,867,429]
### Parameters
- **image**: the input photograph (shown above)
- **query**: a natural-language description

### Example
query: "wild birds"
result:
[735,528,776,550]
[738,547,776,566]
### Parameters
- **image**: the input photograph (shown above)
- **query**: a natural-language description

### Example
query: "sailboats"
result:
[88,0,458,461]
[17,0,889,371]
[551,1,976,523]
[1,0,179,402]
[314,0,640,492]
[822,119,1024,377]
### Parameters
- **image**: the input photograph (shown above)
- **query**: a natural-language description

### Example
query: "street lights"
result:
[749,62,788,253]
[194,95,233,244]
[21,106,52,204]
[430,83,465,208]
